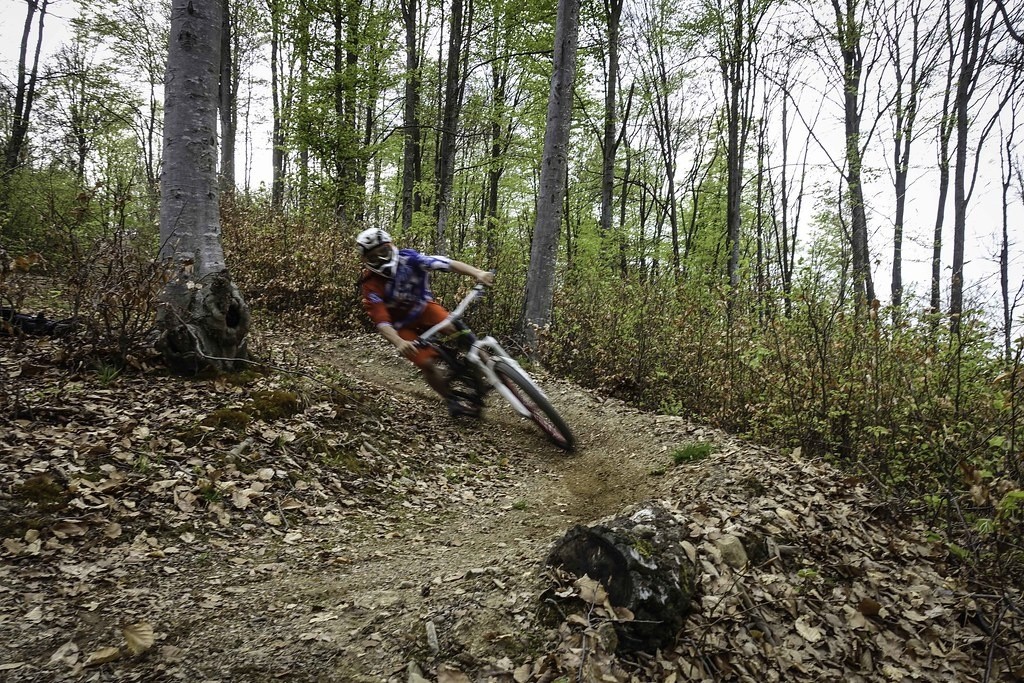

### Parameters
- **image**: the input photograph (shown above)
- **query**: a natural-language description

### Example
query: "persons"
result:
[352,226,498,416]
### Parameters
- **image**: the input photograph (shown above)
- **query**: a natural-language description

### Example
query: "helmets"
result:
[355,227,399,279]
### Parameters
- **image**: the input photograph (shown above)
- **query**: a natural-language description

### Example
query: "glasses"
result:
[361,244,392,266]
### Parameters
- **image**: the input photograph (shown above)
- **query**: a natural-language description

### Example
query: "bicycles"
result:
[391,273,578,459]
